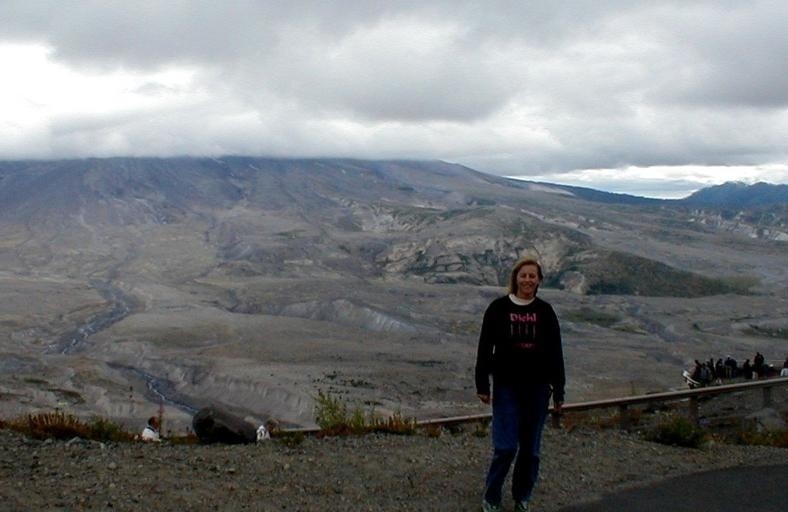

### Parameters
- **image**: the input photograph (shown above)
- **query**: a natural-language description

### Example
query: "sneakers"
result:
[481,499,505,512]
[515,501,531,512]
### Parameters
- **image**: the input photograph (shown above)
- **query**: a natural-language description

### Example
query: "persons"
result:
[256,417,277,442]
[142,415,163,442]
[691,351,788,387]
[475,260,565,512]
